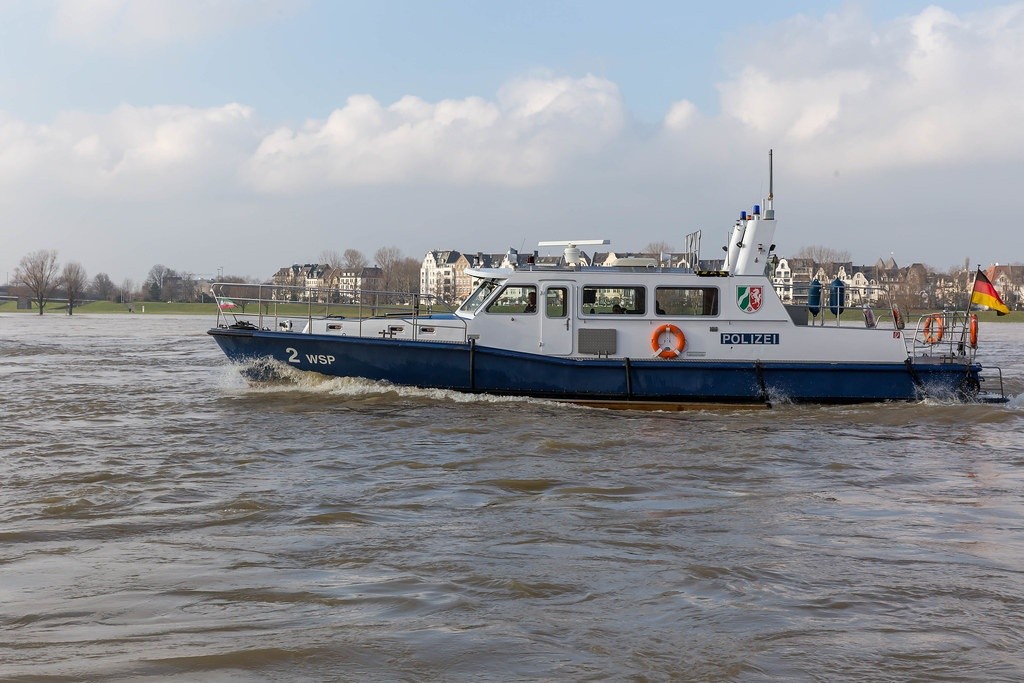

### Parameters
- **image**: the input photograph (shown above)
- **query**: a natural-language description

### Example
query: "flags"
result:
[220,297,237,309]
[970,269,1010,316]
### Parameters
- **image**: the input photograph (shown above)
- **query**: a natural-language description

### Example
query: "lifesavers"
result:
[923,314,943,344]
[969,314,978,347]
[893,307,905,330]
[651,324,685,359]
[863,304,876,328]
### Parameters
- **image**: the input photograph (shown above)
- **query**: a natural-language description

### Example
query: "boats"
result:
[206,145,1013,404]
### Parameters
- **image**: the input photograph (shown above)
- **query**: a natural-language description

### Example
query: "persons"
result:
[656,299,666,315]
[524,292,536,313]
[612,305,629,314]
